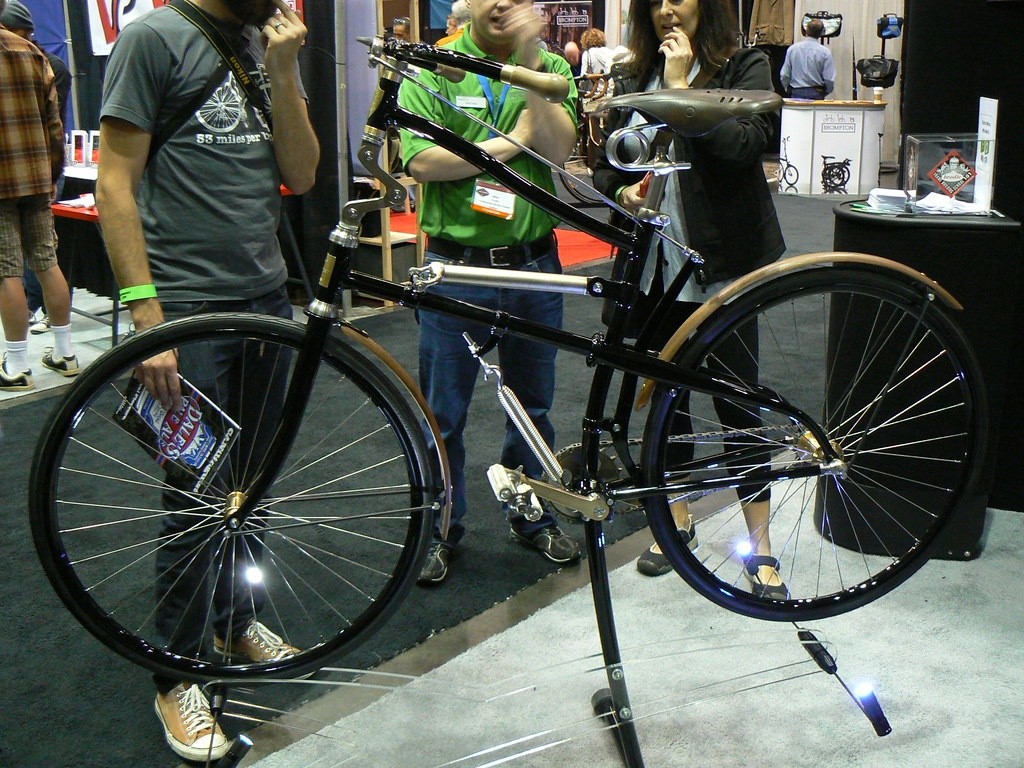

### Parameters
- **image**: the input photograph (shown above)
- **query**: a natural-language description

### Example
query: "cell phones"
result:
[640,171,653,198]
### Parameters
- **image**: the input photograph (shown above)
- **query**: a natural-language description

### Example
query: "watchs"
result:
[615,185,627,205]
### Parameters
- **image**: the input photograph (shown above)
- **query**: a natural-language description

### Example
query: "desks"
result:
[779,98,887,197]
[51,200,130,349]
[814,191,1021,562]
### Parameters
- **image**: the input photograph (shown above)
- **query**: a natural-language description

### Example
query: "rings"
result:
[274,23,283,32]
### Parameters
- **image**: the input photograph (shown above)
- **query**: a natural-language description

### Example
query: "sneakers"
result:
[510,520,581,562]
[638,517,697,576]
[740,549,792,607]
[154,679,229,761]
[29,311,52,333]
[41,347,80,376]
[0,352,36,391]
[214,619,317,680]
[417,544,454,582]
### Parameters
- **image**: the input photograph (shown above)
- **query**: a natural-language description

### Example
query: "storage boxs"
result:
[904,132,997,218]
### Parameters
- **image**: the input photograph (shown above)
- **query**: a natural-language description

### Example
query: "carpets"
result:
[385,207,618,266]
[243,444,1024,768]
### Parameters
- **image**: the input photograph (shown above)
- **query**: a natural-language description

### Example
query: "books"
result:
[112,366,243,499]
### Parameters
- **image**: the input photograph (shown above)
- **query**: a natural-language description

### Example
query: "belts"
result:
[427,232,554,267]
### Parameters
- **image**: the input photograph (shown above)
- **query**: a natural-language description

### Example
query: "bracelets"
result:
[535,63,546,73]
[119,284,159,304]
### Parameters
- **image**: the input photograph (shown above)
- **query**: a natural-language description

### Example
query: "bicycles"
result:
[26,16,989,768]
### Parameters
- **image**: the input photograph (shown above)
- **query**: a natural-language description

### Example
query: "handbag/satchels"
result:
[580,51,597,92]
[602,216,648,340]
[856,55,899,88]
[801,11,842,37]
[877,13,903,39]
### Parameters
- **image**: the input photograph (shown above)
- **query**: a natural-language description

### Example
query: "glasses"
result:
[394,19,406,25]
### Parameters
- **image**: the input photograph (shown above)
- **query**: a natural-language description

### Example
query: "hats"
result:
[0,0,35,33]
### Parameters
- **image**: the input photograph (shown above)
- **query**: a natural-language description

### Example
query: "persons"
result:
[0,0,80,391]
[387,0,836,606]
[95,1,320,763]
